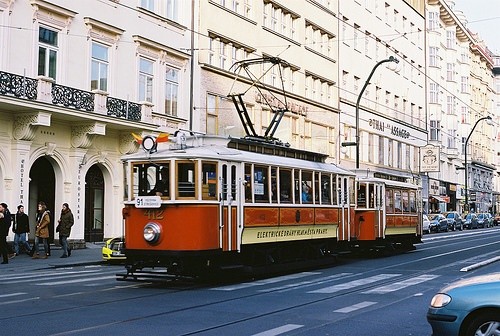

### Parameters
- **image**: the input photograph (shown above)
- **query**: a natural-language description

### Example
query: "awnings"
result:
[432,196,444,203]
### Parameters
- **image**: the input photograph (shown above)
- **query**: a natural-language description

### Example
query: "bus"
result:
[116,57,424,289]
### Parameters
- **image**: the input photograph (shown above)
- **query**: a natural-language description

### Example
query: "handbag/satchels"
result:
[15,214,17,229]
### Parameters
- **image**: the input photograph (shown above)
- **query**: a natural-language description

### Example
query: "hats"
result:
[158,167,168,173]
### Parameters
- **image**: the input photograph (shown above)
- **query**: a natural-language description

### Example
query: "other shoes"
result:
[8,254,14,259]
[32,255,40,258]
[60,255,65,258]
[26,251,33,257]
[47,253,51,256]
[15,253,19,256]
[68,248,71,256]
[40,255,47,259]
[1,261,8,264]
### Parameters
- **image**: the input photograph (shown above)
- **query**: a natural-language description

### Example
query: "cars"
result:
[422,213,431,234]
[426,271,500,336]
[425,213,450,233]
[439,212,464,232]
[460,212,500,230]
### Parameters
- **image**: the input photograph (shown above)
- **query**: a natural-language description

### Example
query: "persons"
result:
[56,203,74,257]
[12,205,31,256]
[26,201,52,259]
[147,167,169,196]
[0,203,12,264]
[302,186,310,202]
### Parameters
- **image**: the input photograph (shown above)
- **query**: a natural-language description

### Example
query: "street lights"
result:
[454,116,493,213]
[341,54,400,169]
